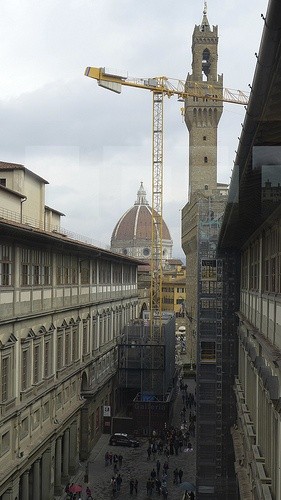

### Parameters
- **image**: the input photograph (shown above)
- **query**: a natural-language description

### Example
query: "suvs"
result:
[110,432,140,447]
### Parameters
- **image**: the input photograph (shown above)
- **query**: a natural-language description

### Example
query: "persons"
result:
[66,372,195,499]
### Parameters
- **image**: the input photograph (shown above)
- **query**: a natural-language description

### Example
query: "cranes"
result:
[84,65,253,401]
